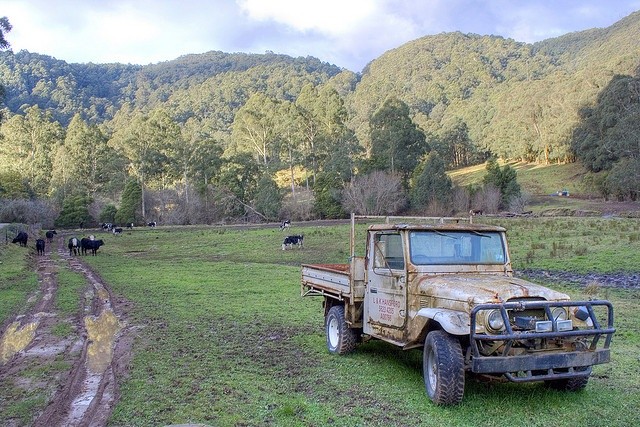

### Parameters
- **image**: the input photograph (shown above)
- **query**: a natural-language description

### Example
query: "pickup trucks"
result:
[299,209,616,408]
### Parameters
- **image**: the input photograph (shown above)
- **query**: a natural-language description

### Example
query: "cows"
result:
[147,222,155,226]
[46,230,57,243]
[36,239,45,255]
[111,228,123,235]
[102,222,117,233]
[12,232,28,247]
[279,219,291,231]
[88,235,95,240]
[81,238,105,257]
[68,237,80,256]
[469,209,481,215]
[281,234,304,251]
[126,222,133,229]
